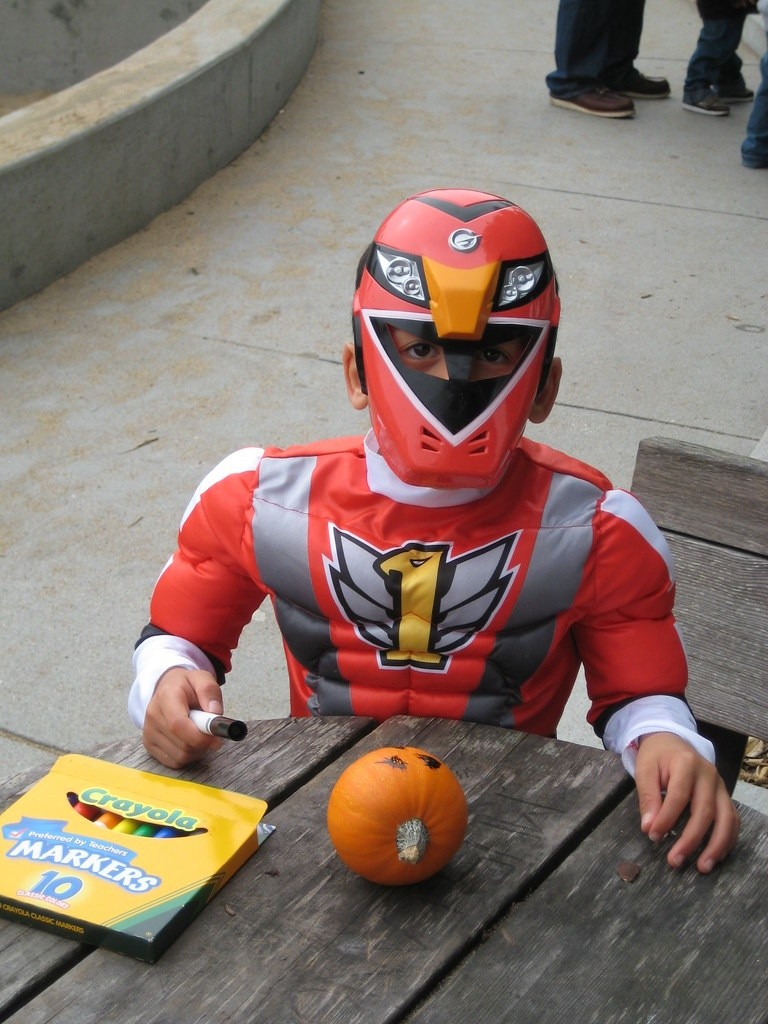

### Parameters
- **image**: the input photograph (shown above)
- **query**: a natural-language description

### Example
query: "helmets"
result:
[350,184,563,492]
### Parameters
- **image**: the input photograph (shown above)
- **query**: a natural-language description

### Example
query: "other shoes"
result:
[606,69,672,99]
[550,86,637,119]
[682,92,731,116]
[718,87,755,102]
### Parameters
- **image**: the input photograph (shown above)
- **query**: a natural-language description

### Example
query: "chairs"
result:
[629,438,768,797]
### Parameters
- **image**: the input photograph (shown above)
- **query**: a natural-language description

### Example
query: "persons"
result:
[544,0,671,118]
[681,0,755,116]
[740,50,768,169]
[126,188,740,870]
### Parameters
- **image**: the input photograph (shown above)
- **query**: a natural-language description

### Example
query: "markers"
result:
[66,790,208,847]
[188,709,248,741]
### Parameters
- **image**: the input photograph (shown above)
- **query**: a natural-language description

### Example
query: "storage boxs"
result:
[0,752,277,963]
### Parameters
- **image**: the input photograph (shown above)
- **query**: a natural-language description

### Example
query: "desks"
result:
[0,718,768,1024]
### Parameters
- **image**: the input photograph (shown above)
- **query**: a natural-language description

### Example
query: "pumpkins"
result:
[327,746,468,884]
